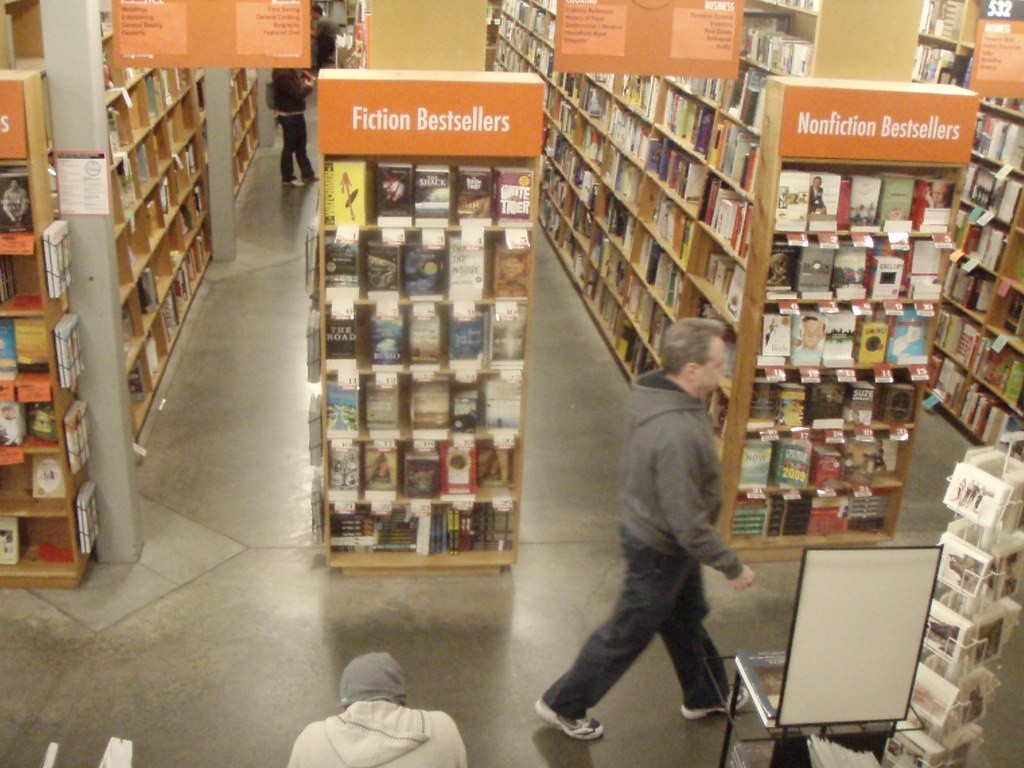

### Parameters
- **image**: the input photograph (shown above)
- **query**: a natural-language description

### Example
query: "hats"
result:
[337,652,407,707]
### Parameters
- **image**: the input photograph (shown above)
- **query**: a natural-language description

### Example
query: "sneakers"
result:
[682,683,750,720]
[534,699,603,739]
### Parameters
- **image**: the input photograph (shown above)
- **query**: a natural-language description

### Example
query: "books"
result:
[324,159,532,226]
[888,442,1024,768]
[324,379,523,436]
[910,43,1024,111]
[0,166,67,566]
[540,68,779,474]
[730,170,954,538]
[98,0,256,402]
[64,399,91,475]
[737,0,818,79]
[43,220,73,299]
[320,233,529,301]
[322,304,525,363]
[326,505,516,554]
[329,442,516,498]
[55,313,85,389]
[493,0,555,80]
[925,114,1024,460]
[76,479,99,554]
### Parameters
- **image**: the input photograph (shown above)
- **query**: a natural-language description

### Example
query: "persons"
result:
[287,652,467,768]
[272,65,322,186]
[309,4,343,72]
[535,319,760,741]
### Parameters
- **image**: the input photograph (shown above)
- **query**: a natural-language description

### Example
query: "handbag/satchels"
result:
[264,84,275,110]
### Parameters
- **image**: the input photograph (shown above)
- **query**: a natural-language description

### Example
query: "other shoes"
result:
[284,179,305,187]
[302,175,319,183]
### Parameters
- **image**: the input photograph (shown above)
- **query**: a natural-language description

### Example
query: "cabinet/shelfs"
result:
[0,1,1024,589]
